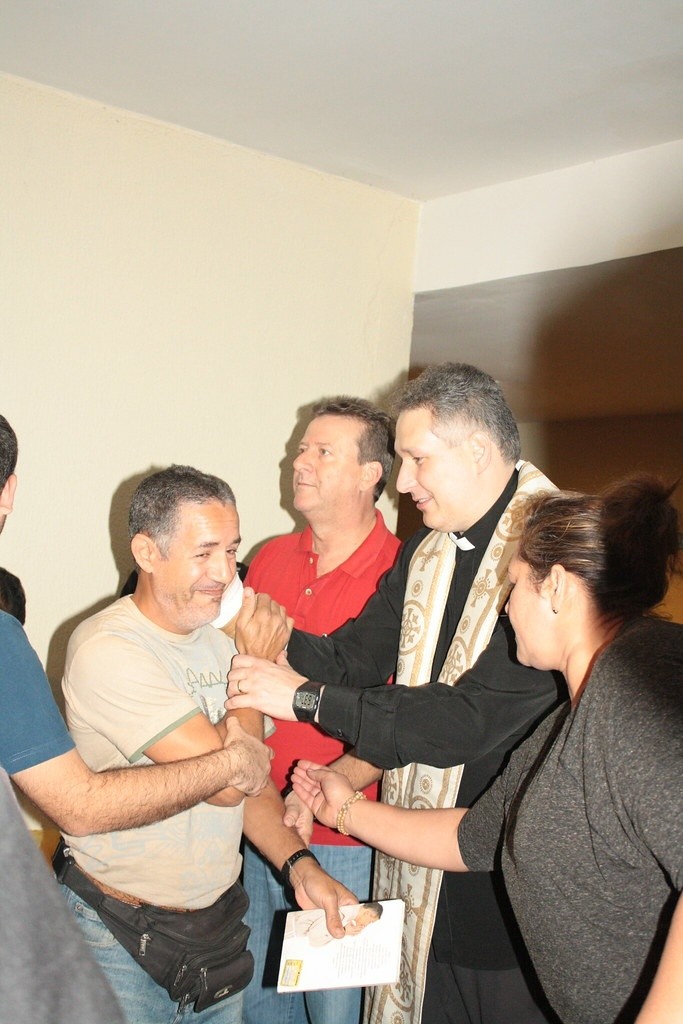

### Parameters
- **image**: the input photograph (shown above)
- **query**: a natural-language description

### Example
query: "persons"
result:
[0,416,274,1024]
[242,397,407,1024]
[292,471,683,1024]
[284,902,383,947]
[49,464,357,1024]
[227,361,573,1024]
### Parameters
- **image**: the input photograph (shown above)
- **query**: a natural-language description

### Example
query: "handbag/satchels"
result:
[49,837,254,1012]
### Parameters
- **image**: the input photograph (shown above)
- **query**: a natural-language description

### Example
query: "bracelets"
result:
[337,791,367,836]
[281,849,321,886]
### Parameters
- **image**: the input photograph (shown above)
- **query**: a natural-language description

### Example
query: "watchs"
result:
[293,681,326,722]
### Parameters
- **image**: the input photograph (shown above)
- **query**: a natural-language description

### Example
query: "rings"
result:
[237,680,245,693]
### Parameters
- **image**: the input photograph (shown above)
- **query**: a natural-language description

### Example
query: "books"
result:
[276,899,406,994]
